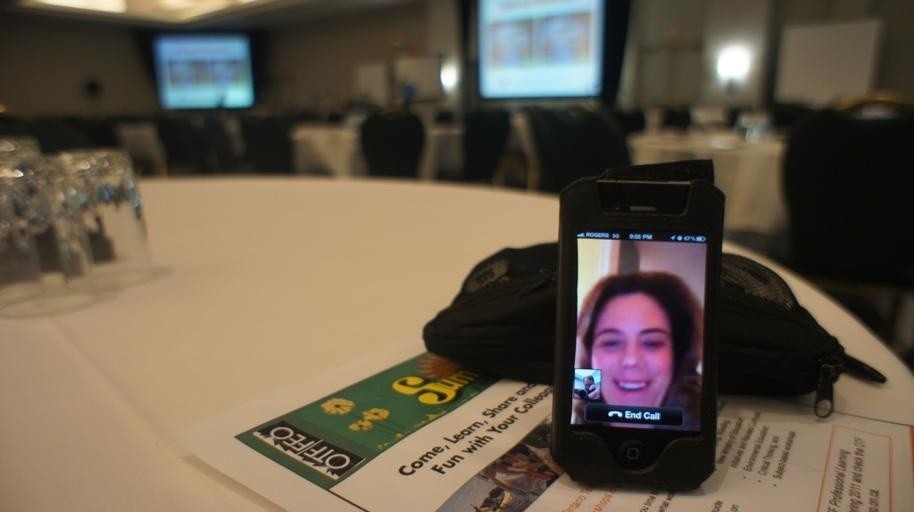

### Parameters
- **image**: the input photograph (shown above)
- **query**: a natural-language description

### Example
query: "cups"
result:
[1,136,155,323]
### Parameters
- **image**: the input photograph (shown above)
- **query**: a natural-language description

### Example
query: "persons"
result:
[575,376,600,400]
[574,272,700,432]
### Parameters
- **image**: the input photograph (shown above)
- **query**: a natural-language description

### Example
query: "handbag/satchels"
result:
[423,243,843,394]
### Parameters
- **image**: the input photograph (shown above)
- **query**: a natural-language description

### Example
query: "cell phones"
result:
[559,177,724,492]
